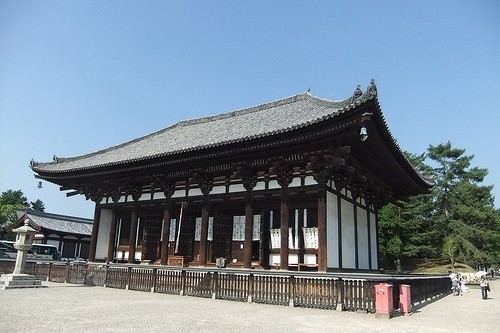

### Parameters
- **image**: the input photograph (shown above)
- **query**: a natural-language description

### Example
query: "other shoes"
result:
[483,297,485,299]
[484,297,487,299]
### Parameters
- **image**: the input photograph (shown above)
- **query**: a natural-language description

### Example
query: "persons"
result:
[497,270,498,275]
[479,275,488,300]
[491,269,494,278]
[448,270,462,296]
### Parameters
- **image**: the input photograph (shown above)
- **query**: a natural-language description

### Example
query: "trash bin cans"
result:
[399,284,412,313]
[374,283,394,313]
[215,257,227,268]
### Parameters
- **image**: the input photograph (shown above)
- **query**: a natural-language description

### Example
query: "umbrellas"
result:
[475,270,488,278]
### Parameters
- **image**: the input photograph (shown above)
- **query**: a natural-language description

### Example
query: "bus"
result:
[0,239,61,262]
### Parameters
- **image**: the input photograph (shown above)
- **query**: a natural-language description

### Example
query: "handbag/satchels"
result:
[484,286,488,290]
